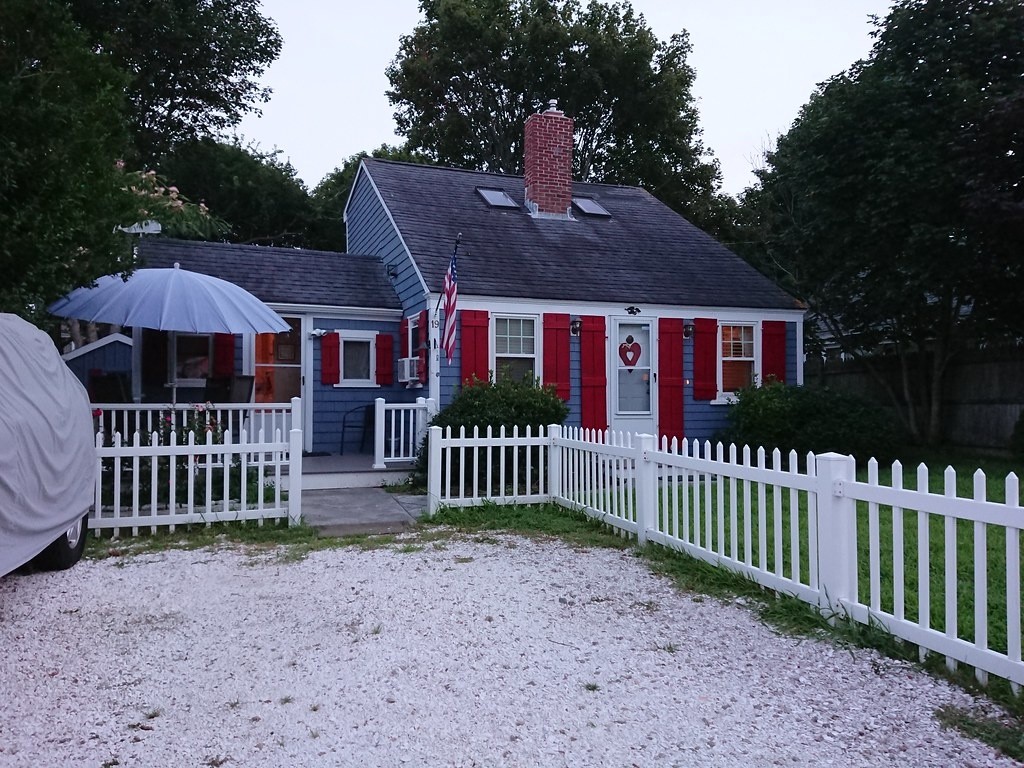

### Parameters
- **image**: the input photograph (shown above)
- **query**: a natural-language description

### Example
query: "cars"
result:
[1,313,99,573]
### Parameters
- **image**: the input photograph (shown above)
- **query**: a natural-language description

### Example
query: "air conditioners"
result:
[397,356,419,382]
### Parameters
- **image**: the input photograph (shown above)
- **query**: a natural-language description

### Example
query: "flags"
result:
[443,248,457,365]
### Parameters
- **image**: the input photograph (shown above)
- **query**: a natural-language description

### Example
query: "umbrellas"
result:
[46,263,293,402]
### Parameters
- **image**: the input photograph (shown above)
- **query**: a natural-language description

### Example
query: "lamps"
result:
[684,321,695,339]
[309,328,328,337]
[570,316,583,329]
